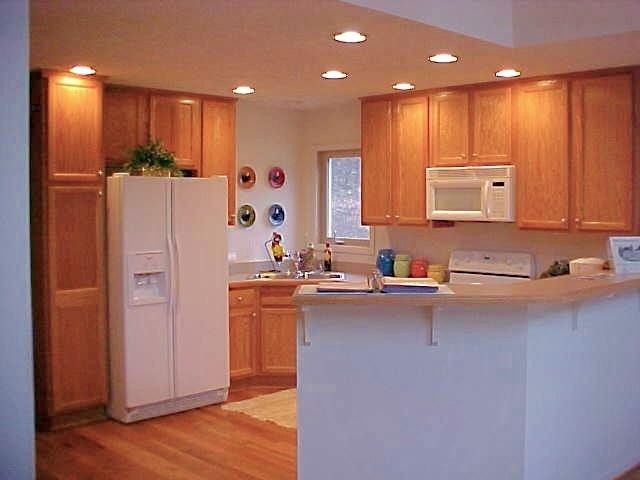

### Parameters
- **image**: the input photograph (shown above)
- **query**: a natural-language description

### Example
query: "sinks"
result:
[303,271,345,282]
[249,273,301,279]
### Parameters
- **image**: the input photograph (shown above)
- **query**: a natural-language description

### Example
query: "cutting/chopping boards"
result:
[315,281,373,292]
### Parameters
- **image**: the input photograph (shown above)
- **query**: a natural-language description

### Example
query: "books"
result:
[315,270,456,297]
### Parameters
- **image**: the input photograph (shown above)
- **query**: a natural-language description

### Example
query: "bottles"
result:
[376,248,448,283]
[323,243,332,271]
[305,241,314,271]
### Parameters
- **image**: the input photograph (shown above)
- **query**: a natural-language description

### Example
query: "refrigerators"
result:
[106,175,231,424]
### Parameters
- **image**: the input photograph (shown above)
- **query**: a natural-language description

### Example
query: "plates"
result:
[238,166,287,228]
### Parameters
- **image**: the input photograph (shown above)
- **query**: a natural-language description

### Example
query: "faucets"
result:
[282,250,304,264]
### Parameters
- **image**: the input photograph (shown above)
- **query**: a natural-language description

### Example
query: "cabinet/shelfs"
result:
[200,98,235,225]
[46,184,106,413]
[512,65,639,236]
[103,86,202,169]
[427,79,513,168]
[359,87,454,228]
[43,74,105,182]
[261,287,296,375]
[228,285,257,379]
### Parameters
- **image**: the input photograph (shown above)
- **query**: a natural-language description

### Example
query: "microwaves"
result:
[425,163,515,225]
